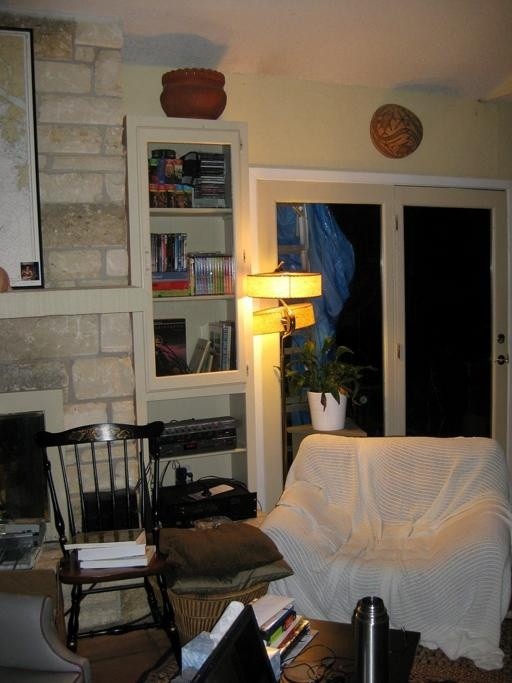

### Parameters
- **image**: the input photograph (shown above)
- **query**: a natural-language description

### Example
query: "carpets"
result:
[137,605,512,683]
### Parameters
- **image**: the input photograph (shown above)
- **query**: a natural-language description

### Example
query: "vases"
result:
[160,68,227,120]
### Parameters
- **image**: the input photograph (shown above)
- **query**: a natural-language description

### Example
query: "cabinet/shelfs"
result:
[123,114,257,507]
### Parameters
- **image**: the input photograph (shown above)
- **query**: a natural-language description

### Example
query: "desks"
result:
[187,617,421,683]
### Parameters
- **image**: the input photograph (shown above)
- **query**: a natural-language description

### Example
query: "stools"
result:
[287,424,369,463]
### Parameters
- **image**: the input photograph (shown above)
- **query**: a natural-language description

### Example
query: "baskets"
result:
[147,576,268,638]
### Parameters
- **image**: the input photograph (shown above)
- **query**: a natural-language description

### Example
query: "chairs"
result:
[37,421,175,654]
[1,569,92,683]
[259,432,512,672]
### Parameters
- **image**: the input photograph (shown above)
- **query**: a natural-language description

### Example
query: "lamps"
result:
[243,261,323,494]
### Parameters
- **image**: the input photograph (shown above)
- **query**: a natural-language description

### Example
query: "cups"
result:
[351,595,389,683]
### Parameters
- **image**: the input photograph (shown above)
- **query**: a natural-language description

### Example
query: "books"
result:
[64,527,156,569]
[241,597,311,660]
[148,149,236,377]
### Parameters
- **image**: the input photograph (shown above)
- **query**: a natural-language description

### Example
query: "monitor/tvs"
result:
[189,605,278,683]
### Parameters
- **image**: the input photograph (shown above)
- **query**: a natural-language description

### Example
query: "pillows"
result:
[158,522,294,595]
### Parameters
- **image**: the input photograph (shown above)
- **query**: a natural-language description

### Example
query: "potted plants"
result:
[272,336,375,432]
